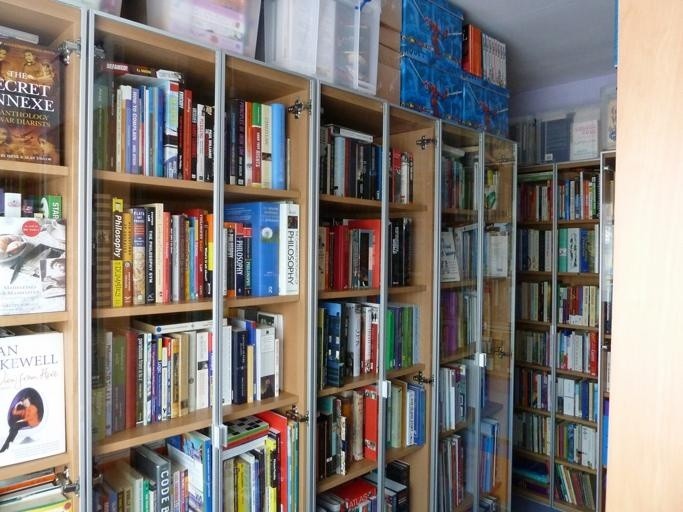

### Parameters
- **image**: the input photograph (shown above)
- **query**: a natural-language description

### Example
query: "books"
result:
[512,100,616,508]
[319,117,426,512]
[439,143,511,511]
[72,0,507,89]
[92,44,213,512]
[223,102,299,512]
[0,26,73,511]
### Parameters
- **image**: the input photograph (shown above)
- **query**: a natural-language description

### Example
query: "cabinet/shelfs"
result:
[512,149,617,512]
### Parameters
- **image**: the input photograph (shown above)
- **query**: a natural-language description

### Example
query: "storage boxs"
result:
[399,42,463,126]
[146,0,263,60]
[461,68,511,139]
[401,1,464,66]
[264,0,382,96]
